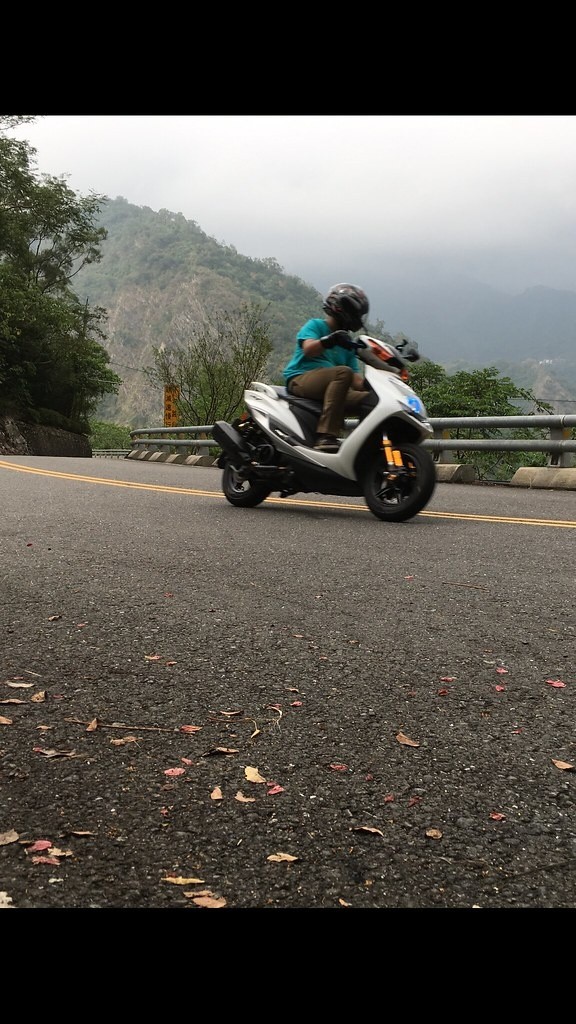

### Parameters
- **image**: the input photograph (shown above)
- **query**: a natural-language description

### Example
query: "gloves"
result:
[320,330,352,351]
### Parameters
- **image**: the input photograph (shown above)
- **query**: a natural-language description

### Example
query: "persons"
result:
[284,283,373,455]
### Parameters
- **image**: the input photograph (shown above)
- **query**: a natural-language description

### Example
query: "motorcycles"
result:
[210,333,438,523]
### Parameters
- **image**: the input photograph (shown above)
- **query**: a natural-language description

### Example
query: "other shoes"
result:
[314,431,343,449]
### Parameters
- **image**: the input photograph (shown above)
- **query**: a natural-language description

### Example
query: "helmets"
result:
[323,283,368,332]
